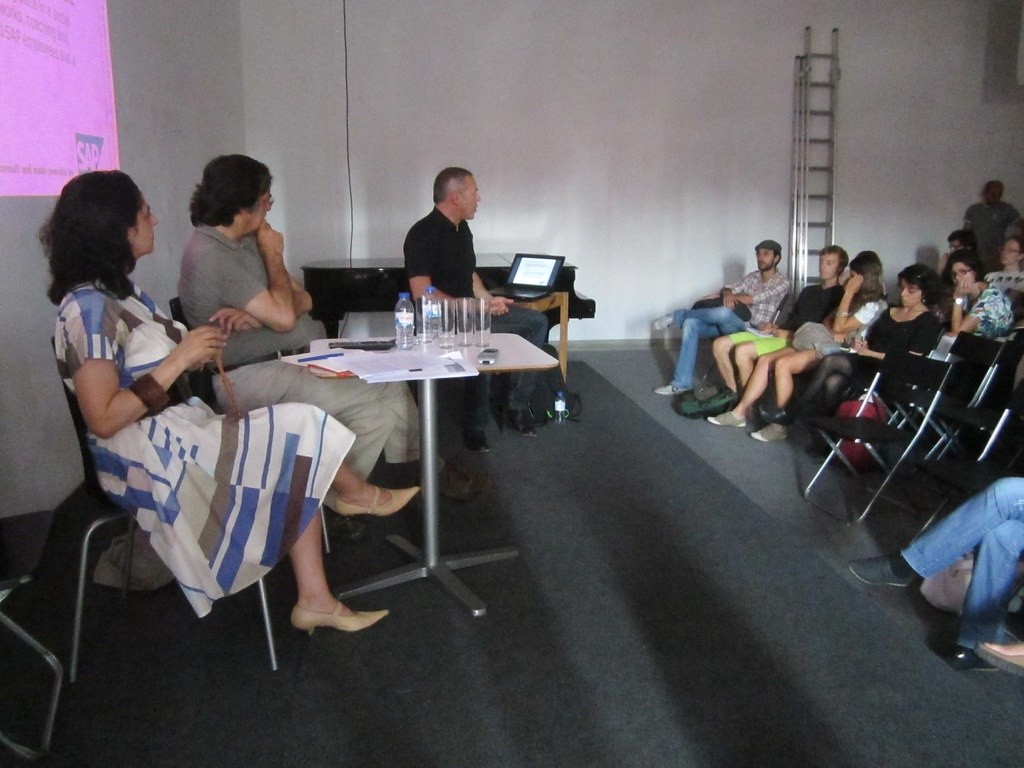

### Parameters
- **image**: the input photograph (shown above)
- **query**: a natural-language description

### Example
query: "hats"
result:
[755,239,782,252]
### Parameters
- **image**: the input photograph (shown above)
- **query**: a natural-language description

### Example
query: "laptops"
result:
[328,336,395,350]
[489,253,565,301]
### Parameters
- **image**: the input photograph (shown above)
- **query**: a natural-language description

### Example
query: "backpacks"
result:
[531,342,582,427]
[835,388,890,469]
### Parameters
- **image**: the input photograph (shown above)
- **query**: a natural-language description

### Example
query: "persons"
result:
[178,155,484,543]
[652,179,1024,678]
[404,167,548,451]
[39,171,420,638]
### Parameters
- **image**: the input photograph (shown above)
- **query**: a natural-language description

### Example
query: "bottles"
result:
[554,391,567,424]
[422,286,441,343]
[394,292,414,349]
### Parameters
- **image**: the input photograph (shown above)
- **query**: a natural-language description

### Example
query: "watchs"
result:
[952,298,963,304]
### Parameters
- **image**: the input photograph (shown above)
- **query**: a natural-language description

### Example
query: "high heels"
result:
[335,486,421,536]
[291,599,390,644]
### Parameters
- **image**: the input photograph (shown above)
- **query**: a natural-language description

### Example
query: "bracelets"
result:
[835,312,848,318]
[129,373,170,415]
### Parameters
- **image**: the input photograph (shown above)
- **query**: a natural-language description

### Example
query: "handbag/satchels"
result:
[691,299,754,322]
[920,550,979,616]
[792,321,842,357]
[92,527,176,591]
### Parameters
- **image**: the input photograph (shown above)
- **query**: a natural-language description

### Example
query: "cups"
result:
[471,297,491,346]
[455,297,475,346]
[436,297,455,348]
[414,296,432,343]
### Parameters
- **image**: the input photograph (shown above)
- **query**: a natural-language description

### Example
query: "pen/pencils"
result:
[298,352,343,362]
[861,336,863,347]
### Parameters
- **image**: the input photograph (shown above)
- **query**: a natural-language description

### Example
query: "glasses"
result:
[949,244,963,251]
[950,269,972,280]
[258,196,275,205]
[998,246,1019,255]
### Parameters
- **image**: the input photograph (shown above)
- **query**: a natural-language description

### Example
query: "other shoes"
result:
[654,385,694,395]
[401,461,494,501]
[948,646,1000,672]
[507,410,538,437]
[974,641,1024,675]
[653,312,673,330]
[751,425,787,442]
[472,431,490,454]
[759,403,796,426]
[849,555,915,587]
[707,412,746,427]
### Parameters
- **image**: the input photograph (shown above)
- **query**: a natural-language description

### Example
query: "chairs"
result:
[51,331,331,685]
[690,280,1024,617]
[169,299,386,557]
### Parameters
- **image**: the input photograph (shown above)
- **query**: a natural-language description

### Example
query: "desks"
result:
[279,334,560,616]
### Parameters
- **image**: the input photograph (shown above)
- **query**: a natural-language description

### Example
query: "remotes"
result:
[476,349,498,365]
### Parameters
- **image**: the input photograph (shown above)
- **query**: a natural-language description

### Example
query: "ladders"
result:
[787,25,840,294]
